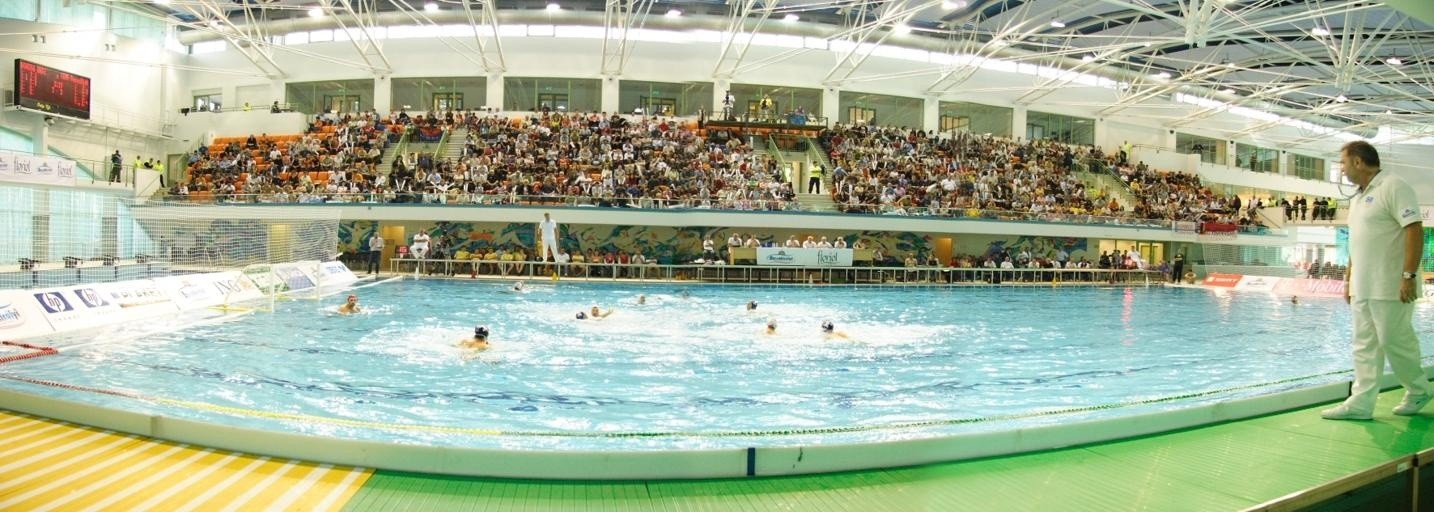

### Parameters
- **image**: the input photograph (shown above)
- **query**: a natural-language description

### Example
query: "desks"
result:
[730,247,873,267]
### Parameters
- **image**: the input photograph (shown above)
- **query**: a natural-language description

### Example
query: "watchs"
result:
[1400,272,1416,281]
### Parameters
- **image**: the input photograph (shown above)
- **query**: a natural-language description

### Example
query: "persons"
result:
[1291,295,1298,304]
[745,300,757,311]
[163,106,1345,284]
[109,150,122,183]
[242,103,252,111]
[133,155,143,168]
[513,282,522,291]
[458,324,489,364]
[1320,140,1434,422]
[765,319,779,336]
[143,157,153,169]
[270,101,281,113]
[337,294,360,316]
[758,93,772,122]
[574,306,613,321]
[639,294,645,305]
[721,90,735,120]
[153,160,165,188]
[794,105,804,117]
[820,320,867,348]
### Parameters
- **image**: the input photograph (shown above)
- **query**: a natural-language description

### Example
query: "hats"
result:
[348,295,358,303]
[822,320,833,331]
[515,282,522,290]
[752,301,757,309]
[576,311,585,319]
[475,325,488,337]
[768,318,777,329]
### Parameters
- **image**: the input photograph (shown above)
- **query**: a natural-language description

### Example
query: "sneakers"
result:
[1393,391,1434,415]
[1321,402,1371,420]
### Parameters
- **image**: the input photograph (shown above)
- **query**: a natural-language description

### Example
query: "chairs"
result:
[177,123,406,204]
